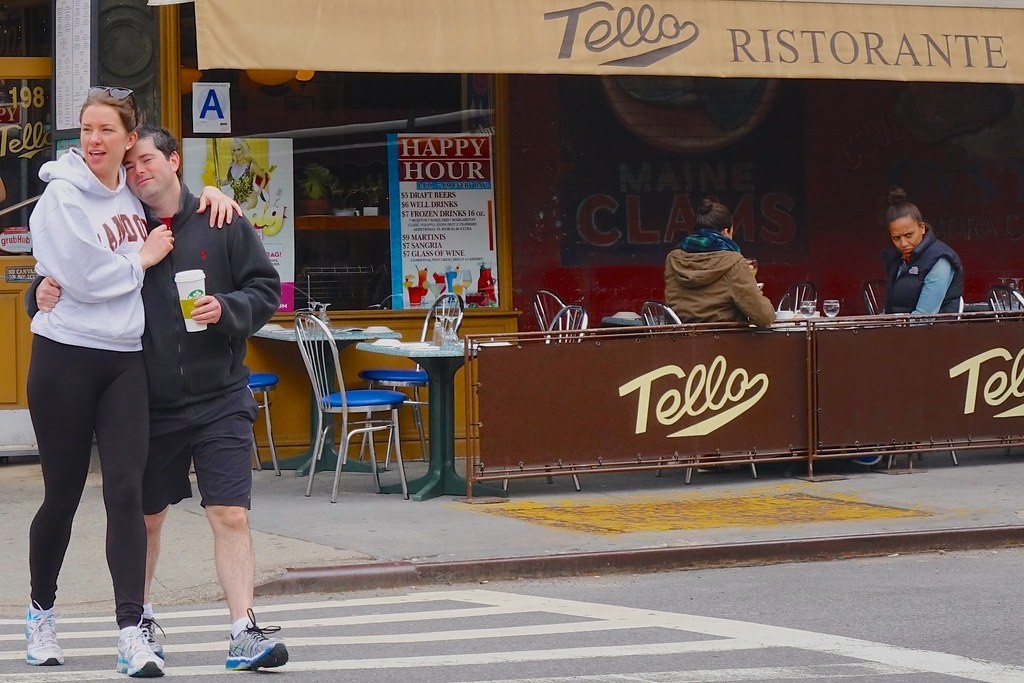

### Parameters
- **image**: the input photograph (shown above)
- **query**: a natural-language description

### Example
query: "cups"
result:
[823,300,840,317]
[175,269,208,334]
[799,301,816,318]
[405,269,498,309]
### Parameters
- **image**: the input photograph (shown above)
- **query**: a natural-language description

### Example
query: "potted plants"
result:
[294,161,337,214]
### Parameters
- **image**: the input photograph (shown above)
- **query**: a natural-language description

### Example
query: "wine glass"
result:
[993,277,1023,312]
[429,283,446,307]
[460,269,472,302]
[451,278,463,308]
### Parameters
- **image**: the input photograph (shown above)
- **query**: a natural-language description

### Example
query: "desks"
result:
[965,303,1012,311]
[252,327,402,477]
[356,341,509,501]
[602,316,666,325]
[774,310,821,322]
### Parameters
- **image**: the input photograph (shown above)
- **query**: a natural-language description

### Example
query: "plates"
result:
[363,325,394,333]
[371,339,406,347]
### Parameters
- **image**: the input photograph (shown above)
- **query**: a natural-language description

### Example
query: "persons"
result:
[664,198,777,327]
[25,124,290,671]
[25,86,244,677]
[851,187,963,465]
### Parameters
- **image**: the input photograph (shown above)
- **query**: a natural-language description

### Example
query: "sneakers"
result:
[24,600,65,666]
[227,608,289,670]
[139,616,165,660]
[851,445,886,465]
[116,615,165,678]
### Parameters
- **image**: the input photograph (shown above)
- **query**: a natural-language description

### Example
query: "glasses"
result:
[86,85,139,129]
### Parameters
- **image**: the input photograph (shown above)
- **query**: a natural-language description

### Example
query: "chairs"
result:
[503,284,1024,490]
[243,291,464,504]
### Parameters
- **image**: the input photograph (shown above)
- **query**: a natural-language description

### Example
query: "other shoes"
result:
[667,460,684,465]
[697,457,714,473]
